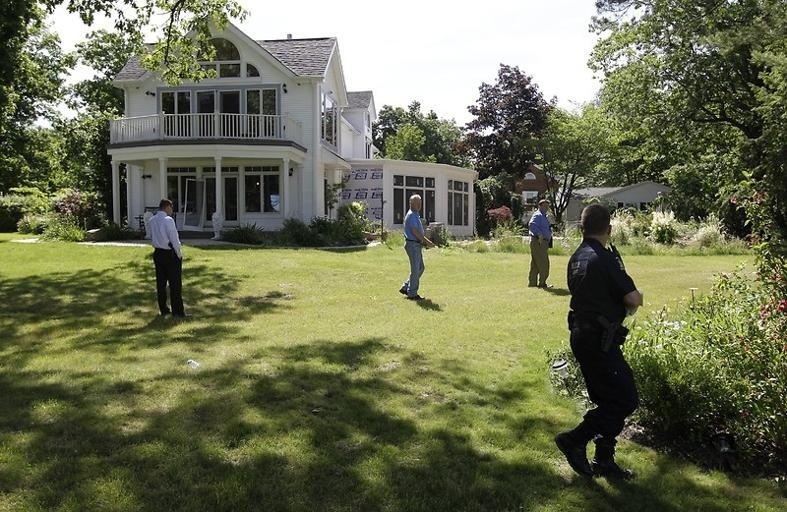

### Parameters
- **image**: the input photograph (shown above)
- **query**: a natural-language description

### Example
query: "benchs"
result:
[139,207,176,231]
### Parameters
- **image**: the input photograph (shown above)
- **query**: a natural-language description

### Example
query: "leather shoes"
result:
[399,289,425,300]
[538,284,553,288]
[184,314,192,317]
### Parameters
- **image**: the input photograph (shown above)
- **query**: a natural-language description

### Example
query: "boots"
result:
[591,443,637,479]
[555,421,593,479]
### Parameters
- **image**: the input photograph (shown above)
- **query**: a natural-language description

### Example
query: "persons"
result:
[149,199,192,318]
[555,202,643,479]
[528,199,553,288]
[399,194,433,301]
